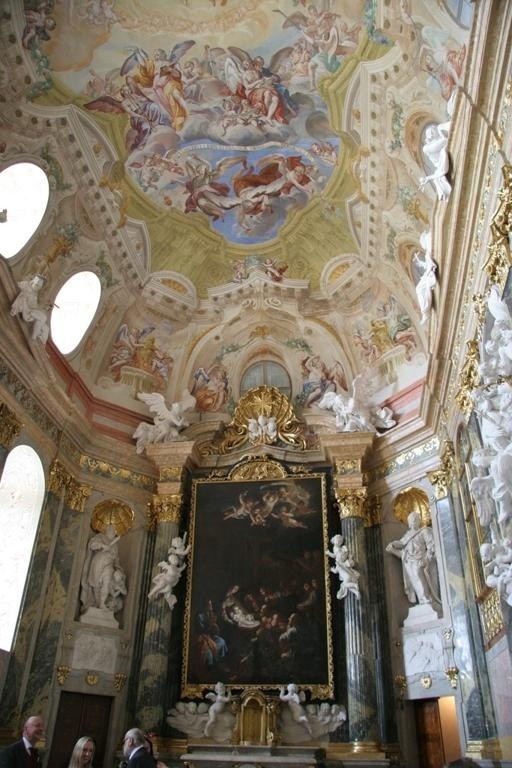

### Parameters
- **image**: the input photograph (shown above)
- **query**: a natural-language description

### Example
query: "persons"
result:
[447,758,482,768]
[123,728,154,768]
[313,749,343,768]
[143,738,168,768]
[0,715,45,768]
[68,736,96,768]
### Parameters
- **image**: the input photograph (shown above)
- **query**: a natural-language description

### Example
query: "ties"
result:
[29,747,38,766]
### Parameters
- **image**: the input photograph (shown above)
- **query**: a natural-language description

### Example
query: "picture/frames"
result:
[179,471,336,702]
